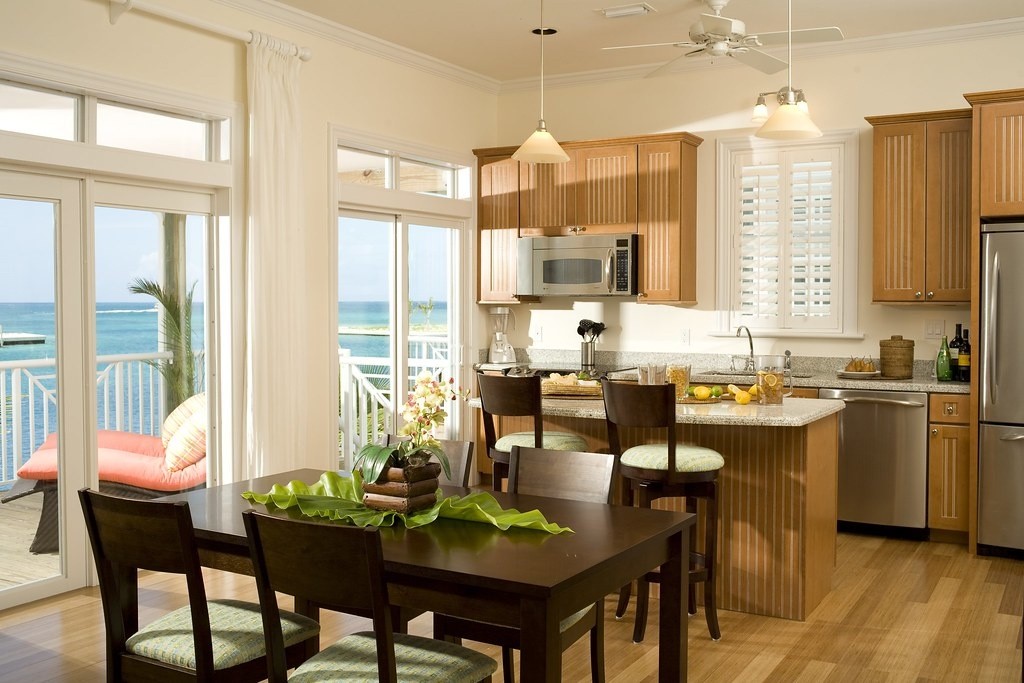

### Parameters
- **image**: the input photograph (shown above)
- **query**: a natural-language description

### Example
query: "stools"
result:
[473,371,589,494]
[598,376,724,641]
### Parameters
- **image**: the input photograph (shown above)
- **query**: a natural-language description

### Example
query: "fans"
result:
[599,0,845,79]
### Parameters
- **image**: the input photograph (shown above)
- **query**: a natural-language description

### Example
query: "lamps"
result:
[750,0,823,140]
[510,0,570,163]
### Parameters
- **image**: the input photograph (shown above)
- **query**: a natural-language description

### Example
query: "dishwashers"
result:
[816,388,928,530]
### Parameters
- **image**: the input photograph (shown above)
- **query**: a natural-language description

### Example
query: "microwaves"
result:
[515,233,636,297]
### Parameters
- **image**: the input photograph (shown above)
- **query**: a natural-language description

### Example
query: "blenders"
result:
[488,306,516,364]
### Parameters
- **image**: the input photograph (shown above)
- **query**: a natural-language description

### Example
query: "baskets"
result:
[541,378,601,395]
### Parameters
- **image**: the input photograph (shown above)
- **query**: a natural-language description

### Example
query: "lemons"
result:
[757,371,777,386]
[688,384,759,405]
[577,372,591,381]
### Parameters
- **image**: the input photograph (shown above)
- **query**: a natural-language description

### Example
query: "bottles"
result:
[936,336,953,380]
[949,324,964,380]
[958,329,971,383]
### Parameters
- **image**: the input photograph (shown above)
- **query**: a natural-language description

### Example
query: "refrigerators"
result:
[978,221,1024,561]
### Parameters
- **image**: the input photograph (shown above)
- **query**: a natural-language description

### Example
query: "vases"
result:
[359,462,443,516]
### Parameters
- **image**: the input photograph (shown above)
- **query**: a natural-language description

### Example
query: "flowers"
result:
[346,375,471,484]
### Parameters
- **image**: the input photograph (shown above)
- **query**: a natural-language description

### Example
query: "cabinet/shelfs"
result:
[929,393,969,545]
[963,86,1024,555]
[471,131,704,307]
[865,107,972,306]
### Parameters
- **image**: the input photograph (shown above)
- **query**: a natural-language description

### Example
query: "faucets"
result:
[736,325,757,370]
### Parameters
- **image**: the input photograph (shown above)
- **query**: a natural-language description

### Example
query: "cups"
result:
[666,364,691,401]
[648,363,666,385]
[757,354,792,407]
[638,363,648,385]
[581,342,594,366]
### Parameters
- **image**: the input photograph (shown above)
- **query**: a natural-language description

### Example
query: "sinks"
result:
[704,370,814,379]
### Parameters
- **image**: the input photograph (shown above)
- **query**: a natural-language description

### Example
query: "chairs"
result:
[0,429,207,553]
[77,487,321,683]
[241,508,498,683]
[433,443,619,683]
[309,434,473,636]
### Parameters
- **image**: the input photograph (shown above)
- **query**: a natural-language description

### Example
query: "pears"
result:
[846,357,877,372]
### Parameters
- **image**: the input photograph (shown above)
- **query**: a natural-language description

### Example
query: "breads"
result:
[542,372,578,386]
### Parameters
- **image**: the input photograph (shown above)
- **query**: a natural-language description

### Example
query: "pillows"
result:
[161,391,206,447]
[164,406,207,470]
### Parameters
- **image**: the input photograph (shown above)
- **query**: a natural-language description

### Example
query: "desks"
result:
[153,469,700,683]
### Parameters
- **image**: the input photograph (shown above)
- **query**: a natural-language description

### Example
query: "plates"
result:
[838,370,881,377]
